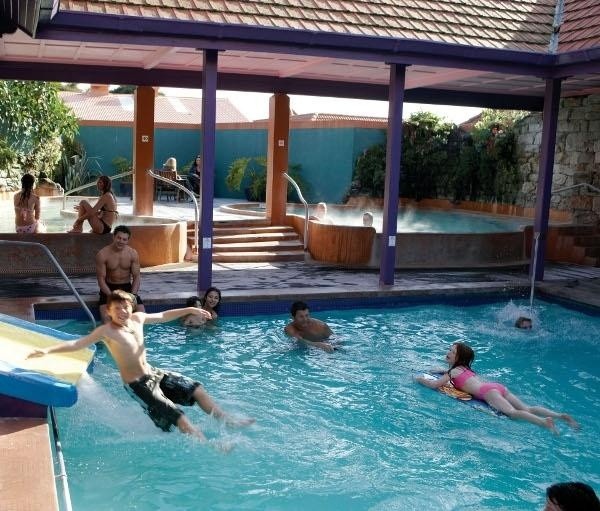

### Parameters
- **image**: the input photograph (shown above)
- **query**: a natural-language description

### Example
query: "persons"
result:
[363,212,373,227]
[415,342,578,436]
[23,289,256,455]
[316,202,334,225]
[542,481,600,511]
[514,317,532,330]
[96,225,145,322]
[179,287,221,324]
[67,176,117,235]
[284,301,335,353]
[14,174,40,233]
[183,296,218,337]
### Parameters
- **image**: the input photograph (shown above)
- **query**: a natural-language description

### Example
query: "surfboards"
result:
[412,366,513,420]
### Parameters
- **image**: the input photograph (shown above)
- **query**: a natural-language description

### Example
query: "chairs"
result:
[154,169,185,204]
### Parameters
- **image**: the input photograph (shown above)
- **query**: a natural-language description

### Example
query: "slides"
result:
[0,315,97,408]
